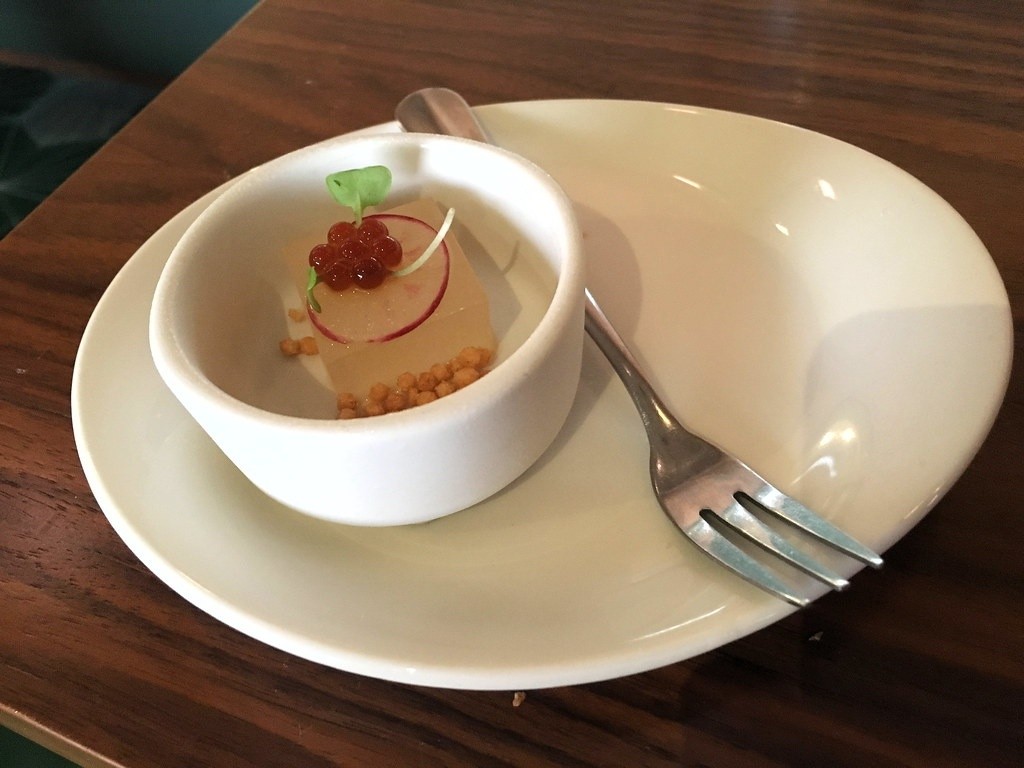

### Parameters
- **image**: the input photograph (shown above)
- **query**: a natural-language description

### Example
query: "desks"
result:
[0,0,1024,768]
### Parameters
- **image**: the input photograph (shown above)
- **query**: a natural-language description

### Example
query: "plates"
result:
[71,98,1015,689]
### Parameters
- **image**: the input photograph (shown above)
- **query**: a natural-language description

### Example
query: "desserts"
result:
[286,197,496,408]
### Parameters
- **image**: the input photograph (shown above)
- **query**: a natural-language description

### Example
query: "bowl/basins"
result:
[150,130,587,527]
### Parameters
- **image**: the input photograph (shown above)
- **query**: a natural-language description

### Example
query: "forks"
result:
[397,87,885,608]
